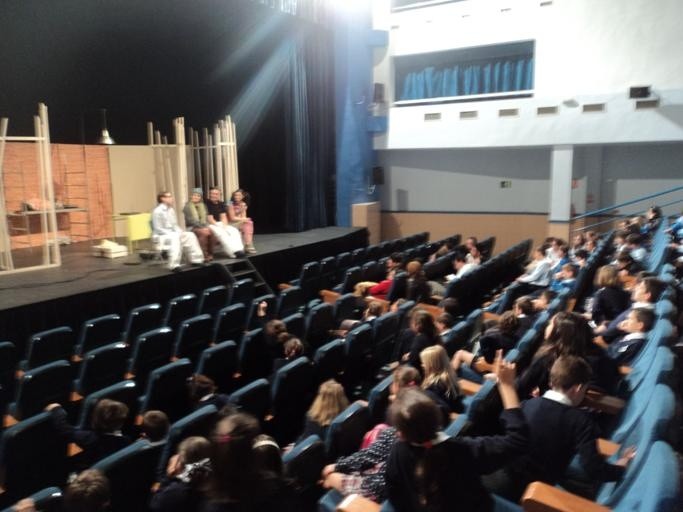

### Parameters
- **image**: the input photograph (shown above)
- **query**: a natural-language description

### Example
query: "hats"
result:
[191,188,204,196]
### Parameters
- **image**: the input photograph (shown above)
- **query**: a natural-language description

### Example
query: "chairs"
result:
[0,211,683,512]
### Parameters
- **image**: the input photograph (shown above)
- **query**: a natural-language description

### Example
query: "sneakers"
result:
[204,246,257,263]
[172,267,182,273]
[192,261,205,267]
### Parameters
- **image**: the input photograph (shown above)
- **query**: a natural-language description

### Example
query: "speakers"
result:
[630,86,651,98]
[372,165,385,186]
[374,83,386,103]
